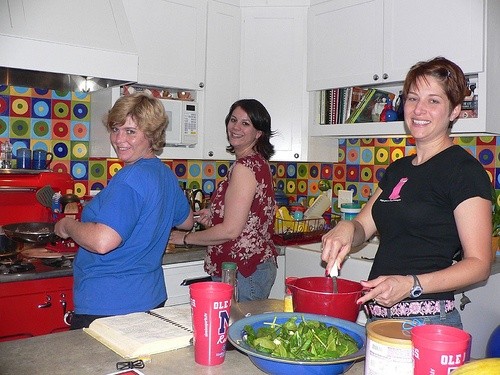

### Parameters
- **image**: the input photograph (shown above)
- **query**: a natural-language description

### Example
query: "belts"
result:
[362,298,455,319]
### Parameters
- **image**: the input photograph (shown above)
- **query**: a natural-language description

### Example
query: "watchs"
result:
[410,275,423,299]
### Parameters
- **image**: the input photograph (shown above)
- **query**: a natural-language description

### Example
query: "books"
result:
[327,88,391,124]
[83,301,233,361]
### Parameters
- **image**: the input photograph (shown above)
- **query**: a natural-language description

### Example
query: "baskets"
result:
[273,212,342,239]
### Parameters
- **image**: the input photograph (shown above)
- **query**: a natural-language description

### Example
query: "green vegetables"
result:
[241,314,358,360]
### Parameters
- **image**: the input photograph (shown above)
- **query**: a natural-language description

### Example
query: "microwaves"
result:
[156,98,199,147]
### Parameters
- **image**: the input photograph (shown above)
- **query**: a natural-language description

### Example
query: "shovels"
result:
[36,185,55,208]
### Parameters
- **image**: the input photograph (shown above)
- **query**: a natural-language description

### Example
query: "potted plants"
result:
[491,191,500,260]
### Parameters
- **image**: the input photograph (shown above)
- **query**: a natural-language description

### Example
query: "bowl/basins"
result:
[227,312,367,375]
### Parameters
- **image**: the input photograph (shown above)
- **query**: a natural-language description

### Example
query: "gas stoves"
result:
[0,233,35,277]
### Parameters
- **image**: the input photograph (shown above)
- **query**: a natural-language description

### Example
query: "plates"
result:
[20,248,73,258]
[173,97,194,101]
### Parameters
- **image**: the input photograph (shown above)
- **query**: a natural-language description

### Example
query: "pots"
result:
[1,221,60,245]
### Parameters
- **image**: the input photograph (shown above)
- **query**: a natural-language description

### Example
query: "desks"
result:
[0,296,367,375]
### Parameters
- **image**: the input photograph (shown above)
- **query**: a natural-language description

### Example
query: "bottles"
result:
[0,139,12,168]
[220,262,246,351]
[340,203,361,223]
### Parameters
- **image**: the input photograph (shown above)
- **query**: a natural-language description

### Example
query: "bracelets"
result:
[184,231,192,249]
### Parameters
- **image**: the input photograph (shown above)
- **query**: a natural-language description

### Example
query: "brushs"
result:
[304,195,331,218]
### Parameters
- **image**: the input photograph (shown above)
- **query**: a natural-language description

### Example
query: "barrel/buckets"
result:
[364,318,417,375]
[285,276,370,323]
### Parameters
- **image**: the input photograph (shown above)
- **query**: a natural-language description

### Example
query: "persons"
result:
[320,57,493,330]
[53,93,195,331]
[167,99,279,302]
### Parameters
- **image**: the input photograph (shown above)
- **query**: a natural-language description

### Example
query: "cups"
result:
[178,91,191,98]
[189,282,234,366]
[411,324,471,375]
[16,148,31,169]
[32,149,53,169]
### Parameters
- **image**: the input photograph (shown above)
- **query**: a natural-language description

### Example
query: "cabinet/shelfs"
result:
[0,237,382,342]
[0,0,500,163]
